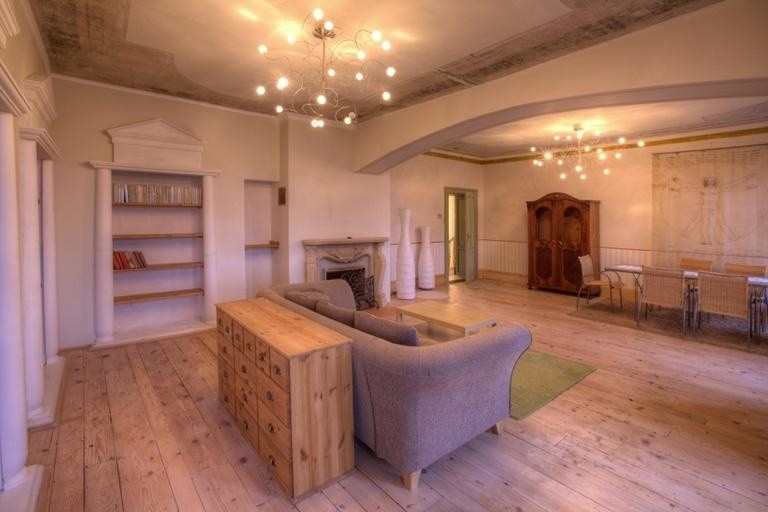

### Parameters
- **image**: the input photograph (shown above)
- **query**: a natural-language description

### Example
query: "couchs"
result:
[256,279,533,490]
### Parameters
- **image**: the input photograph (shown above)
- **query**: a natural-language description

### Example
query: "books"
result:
[111,181,202,206]
[113,250,146,270]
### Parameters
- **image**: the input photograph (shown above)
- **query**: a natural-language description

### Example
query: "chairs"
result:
[576,253,624,307]
[638,258,767,349]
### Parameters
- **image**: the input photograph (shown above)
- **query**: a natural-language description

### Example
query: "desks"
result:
[601,262,767,344]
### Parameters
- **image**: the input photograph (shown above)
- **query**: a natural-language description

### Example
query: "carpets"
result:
[566,295,768,357]
[507,349,597,424]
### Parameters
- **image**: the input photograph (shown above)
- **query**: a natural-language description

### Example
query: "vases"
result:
[417,225,437,289]
[397,208,415,300]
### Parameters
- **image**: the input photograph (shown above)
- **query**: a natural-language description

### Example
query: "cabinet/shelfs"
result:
[111,200,206,305]
[213,298,358,506]
[525,192,600,298]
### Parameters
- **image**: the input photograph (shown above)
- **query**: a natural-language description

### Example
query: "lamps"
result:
[254,5,399,128]
